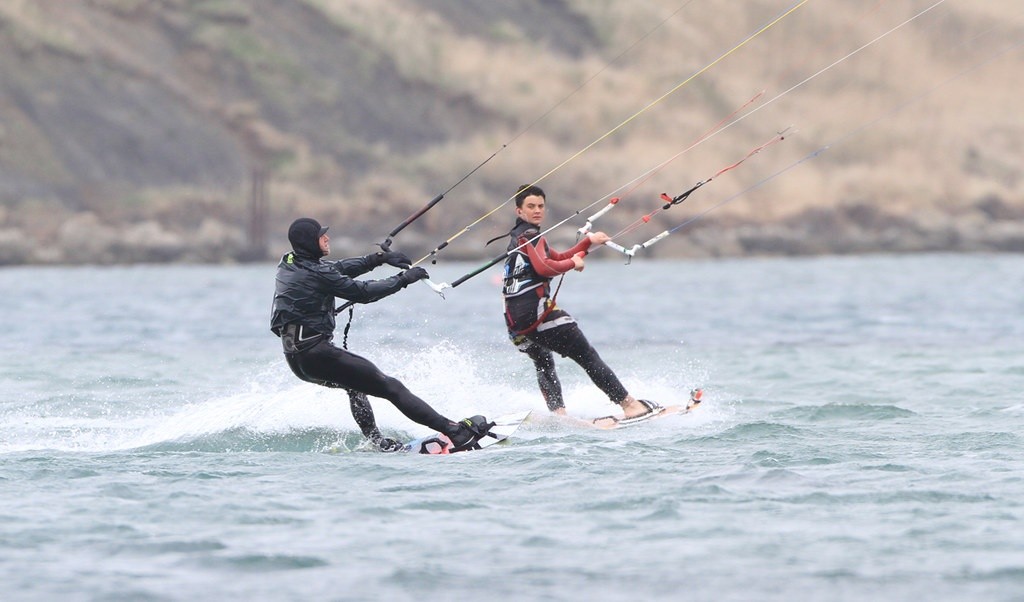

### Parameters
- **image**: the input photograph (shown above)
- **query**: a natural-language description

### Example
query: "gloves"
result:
[376,251,412,270]
[401,266,430,288]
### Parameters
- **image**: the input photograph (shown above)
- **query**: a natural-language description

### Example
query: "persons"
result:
[269,218,487,448]
[502,184,663,417]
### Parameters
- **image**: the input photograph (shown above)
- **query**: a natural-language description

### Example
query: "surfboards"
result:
[399,407,533,455]
[591,402,699,430]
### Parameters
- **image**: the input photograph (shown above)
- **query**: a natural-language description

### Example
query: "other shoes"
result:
[450,415,488,448]
[375,439,403,455]
